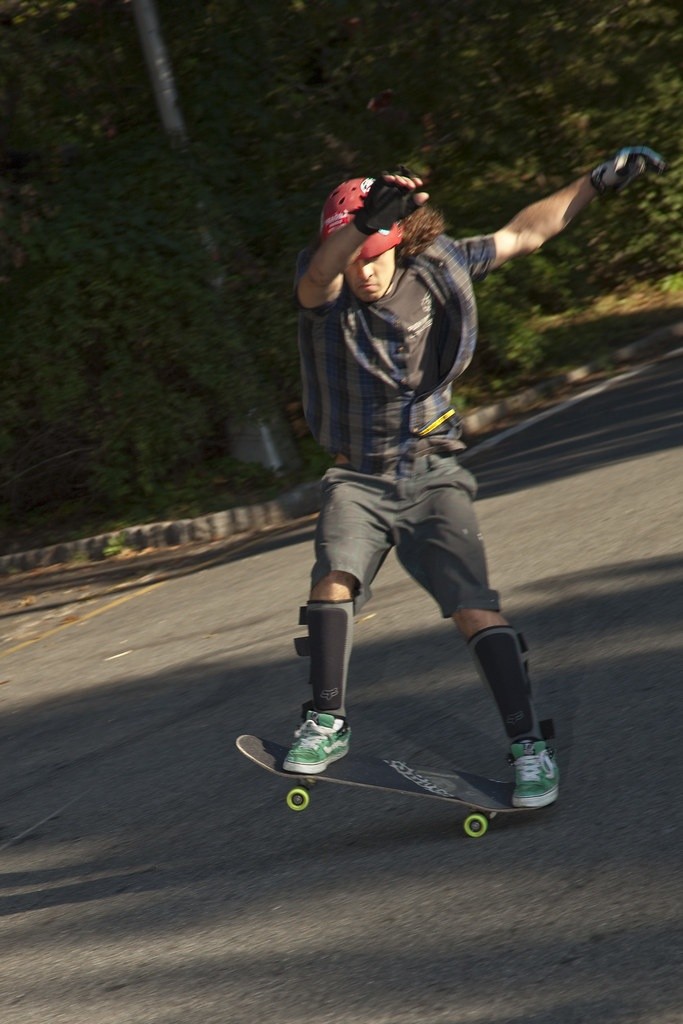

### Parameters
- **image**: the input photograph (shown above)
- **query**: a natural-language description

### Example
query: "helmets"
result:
[320,178,403,260]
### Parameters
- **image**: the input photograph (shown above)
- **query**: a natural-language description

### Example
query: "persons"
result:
[283,148,667,809]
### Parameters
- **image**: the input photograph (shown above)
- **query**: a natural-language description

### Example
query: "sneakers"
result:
[283,710,350,774]
[505,740,559,807]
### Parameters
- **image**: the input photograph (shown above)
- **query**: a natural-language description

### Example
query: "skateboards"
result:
[231,730,557,841]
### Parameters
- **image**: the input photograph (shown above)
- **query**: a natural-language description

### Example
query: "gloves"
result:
[590,146,665,195]
